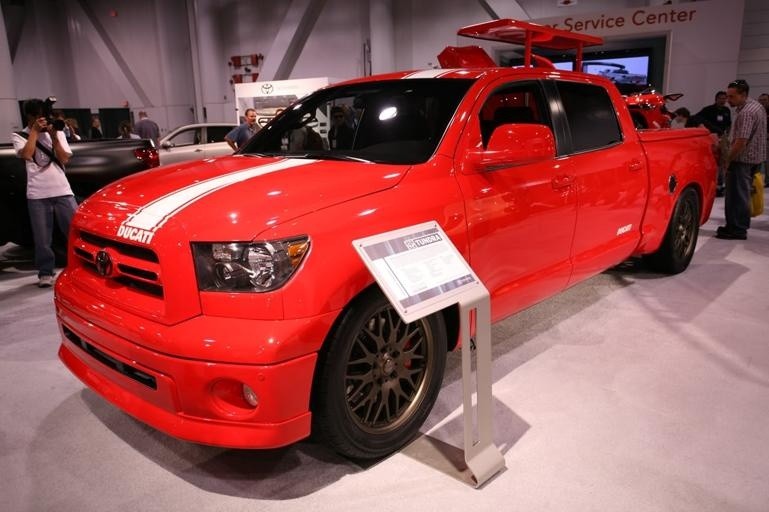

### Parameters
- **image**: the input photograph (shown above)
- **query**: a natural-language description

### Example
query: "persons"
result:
[671,79,769,240]
[11,95,82,289]
[55,107,162,151]
[225,102,363,157]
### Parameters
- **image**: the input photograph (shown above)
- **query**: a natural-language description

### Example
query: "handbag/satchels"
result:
[751,173,764,216]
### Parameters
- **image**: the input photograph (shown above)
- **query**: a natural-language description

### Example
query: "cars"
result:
[154,122,240,166]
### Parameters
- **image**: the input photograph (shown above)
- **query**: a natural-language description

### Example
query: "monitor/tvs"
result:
[508,47,655,129]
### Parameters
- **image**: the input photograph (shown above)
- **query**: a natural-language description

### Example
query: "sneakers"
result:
[716,226,747,240]
[38,275,53,287]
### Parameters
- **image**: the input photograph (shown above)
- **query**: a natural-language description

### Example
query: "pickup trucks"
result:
[52,17,717,463]
[614,82,684,130]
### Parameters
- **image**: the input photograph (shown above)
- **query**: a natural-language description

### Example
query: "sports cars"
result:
[0,132,160,264]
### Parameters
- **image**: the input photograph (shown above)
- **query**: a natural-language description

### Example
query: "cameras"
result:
[40,96,66,131]
